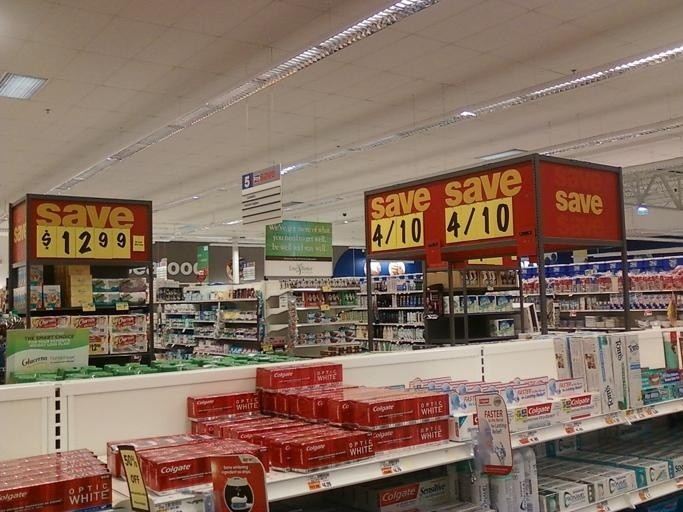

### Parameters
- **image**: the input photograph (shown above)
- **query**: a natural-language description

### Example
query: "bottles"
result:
[513,297,560,310]
[398,325,425,340]
[456,446,540,512]
[230,288,255,298]
[618,276,674,290]
[373,325,398,339]
[521,274,612,292]
[397,293,423,307]
[560,295,611,309]
[373,340,391,351]
[391,341,413,351]
[373,309,424,323]
[610,292,683,309]
[356,295,392,307]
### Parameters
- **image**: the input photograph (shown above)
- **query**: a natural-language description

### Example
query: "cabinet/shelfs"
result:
[263,278,362,358]
[525,290,683,329]
[356,290,424,343]
[0,328,683,512]
[422,261,523,342]
[8,264,154,365]
[154,297,258,357]
